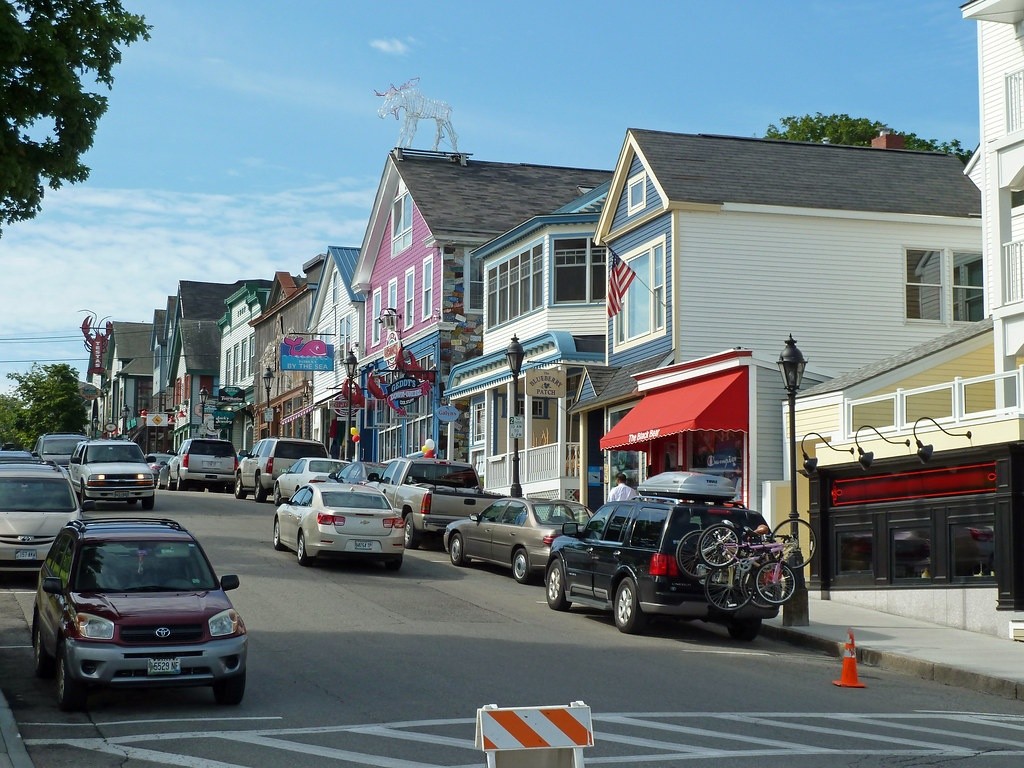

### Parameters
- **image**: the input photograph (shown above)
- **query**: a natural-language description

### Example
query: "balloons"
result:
[422,439,435,458]
[351,427,359,442]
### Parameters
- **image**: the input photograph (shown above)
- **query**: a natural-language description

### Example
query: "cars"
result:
[325,462,390,485]
[443,497,596,585]
[273,482,406,571]
[144,452,175,490]
[273,457,359,507]
[0,450,99,572]
[839,523,996,579]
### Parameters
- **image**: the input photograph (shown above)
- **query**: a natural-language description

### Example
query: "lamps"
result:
[378,308,401,323]
[855,425,910,471]
[801,433,854,475]
[913,417,971,465]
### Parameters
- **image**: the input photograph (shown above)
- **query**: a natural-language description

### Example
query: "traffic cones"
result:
[833,628,868,688]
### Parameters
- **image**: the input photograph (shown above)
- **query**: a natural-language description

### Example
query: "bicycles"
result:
[676,517,819,611]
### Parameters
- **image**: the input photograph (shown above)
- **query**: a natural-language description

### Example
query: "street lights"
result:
[343,348,359,463]
[777,332,811,627]
[92,415,100,439]
[261,364,275,438]
[198,385,210,438]
[504,332,525,498]
[121,404,130,435]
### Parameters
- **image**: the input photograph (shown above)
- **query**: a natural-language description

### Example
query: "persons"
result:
[606,473,636,502]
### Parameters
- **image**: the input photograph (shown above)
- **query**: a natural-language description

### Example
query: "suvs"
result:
[543,471,785,644]
[234,435,334,503]
[68,436,157,511]
[30,432,94,477]
[31,517,249,713]
[166,437,239,494]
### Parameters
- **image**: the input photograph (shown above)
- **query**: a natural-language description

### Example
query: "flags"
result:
[606,248,636,320]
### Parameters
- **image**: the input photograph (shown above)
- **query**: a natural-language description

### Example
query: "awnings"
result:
[600,371,749,452]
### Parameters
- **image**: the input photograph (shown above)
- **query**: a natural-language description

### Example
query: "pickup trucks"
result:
[364,458,513,550]
